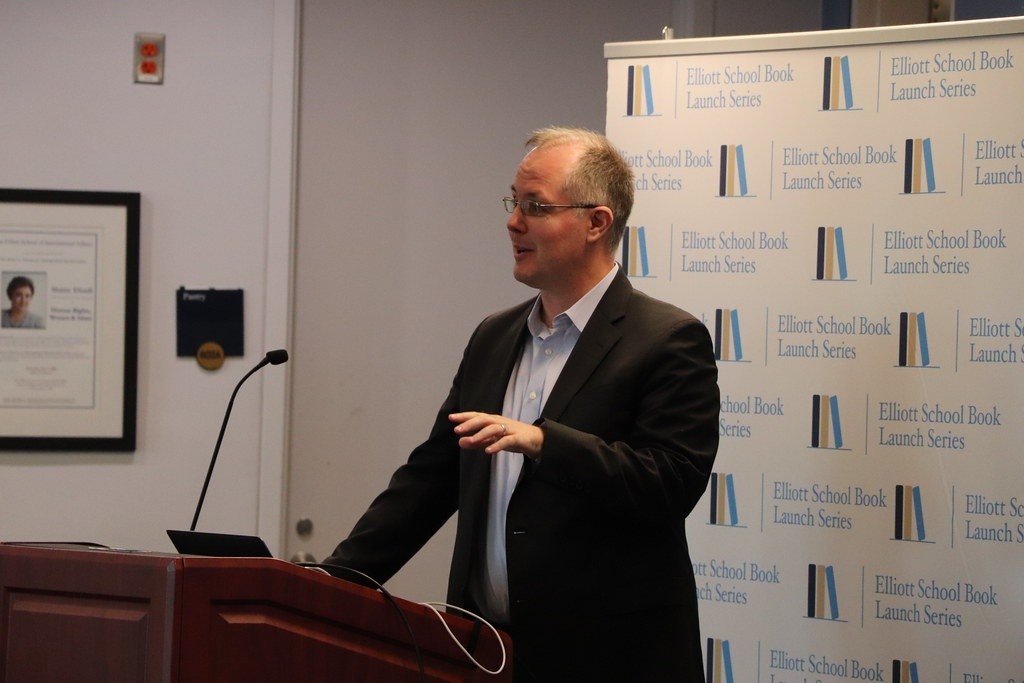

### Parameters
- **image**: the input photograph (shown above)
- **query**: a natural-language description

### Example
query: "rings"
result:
[501,424,506,435]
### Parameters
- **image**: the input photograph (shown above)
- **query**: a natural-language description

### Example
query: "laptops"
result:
[166,529,274,559]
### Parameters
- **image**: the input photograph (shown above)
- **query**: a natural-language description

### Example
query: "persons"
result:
[317,123,721,683]
[0,276,43,328]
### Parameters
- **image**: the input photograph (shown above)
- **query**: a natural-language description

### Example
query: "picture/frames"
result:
[0,188,142,457]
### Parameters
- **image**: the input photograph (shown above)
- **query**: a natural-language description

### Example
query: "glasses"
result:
[499,195,598,219]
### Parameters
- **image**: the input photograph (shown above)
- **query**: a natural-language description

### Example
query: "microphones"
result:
[189,349,289,530]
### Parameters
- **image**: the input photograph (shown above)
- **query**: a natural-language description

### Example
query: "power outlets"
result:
[132,32,166,86]
